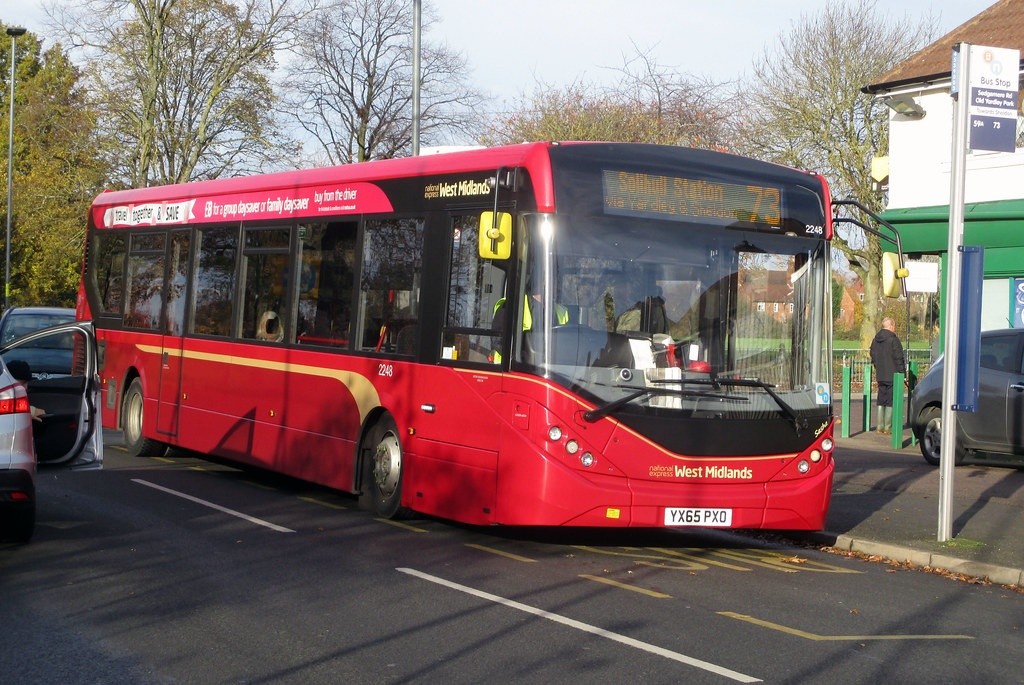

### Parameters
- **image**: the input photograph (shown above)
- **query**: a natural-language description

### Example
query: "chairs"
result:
[297,313,417,355]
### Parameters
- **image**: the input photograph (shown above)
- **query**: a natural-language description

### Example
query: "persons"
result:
[870,317,906,433]
[5,360,47,423]
[490,266,568,366]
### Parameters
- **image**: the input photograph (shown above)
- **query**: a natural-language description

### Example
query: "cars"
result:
[0,319,105,530]
[1,306,79,374]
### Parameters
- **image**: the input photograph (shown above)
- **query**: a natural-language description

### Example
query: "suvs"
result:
[910,327,1024,467]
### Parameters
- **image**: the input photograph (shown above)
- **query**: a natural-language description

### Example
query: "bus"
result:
[72,143,909,532]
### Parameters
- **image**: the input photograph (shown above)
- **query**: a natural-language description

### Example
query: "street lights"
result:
[5,27,28,313]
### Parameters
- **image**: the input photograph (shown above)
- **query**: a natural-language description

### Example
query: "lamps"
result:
[883,96,926,118]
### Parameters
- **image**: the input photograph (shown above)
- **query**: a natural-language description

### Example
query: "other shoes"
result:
[876,430,892,435]
